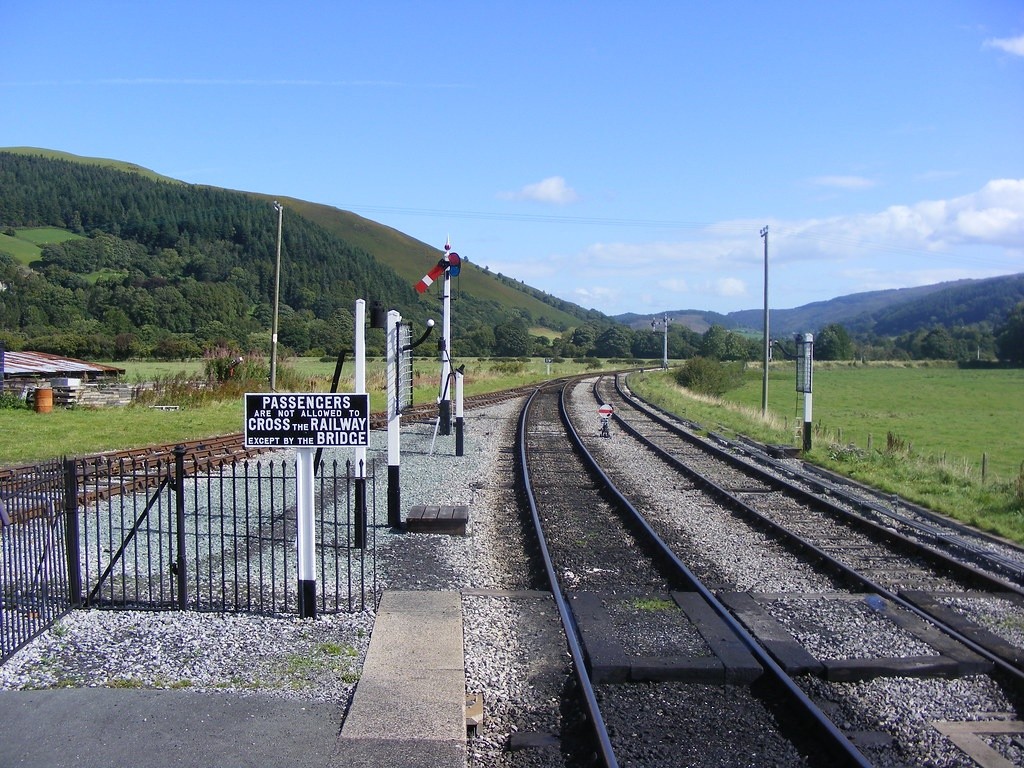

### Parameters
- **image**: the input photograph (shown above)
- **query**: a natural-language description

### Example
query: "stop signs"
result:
[598,404,615,419]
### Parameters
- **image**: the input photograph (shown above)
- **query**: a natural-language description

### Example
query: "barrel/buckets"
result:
[35,387,53,413]
